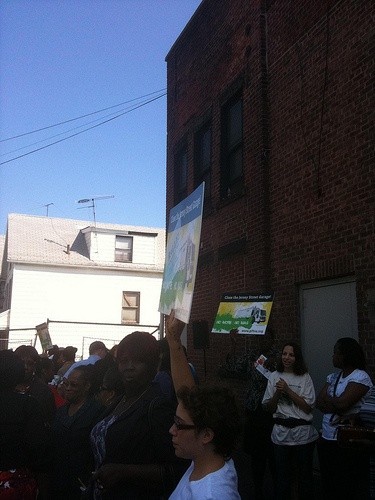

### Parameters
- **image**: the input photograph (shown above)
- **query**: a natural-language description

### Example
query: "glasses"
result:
[100,385,114,392]
[174,416,196,430]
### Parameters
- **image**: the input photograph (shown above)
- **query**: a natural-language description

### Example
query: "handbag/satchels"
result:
[335,425,374,454]
